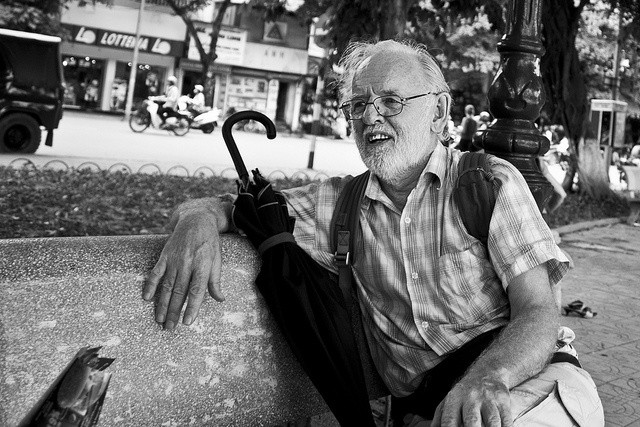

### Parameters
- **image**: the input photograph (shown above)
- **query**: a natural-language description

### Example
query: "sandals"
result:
[565,300,597,319]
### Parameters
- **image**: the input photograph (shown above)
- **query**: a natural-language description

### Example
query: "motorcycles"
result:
[174,100,222,134]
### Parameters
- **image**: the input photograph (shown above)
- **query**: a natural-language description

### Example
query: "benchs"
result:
[0,233,393,426]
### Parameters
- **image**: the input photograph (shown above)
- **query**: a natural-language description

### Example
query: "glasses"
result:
[338,94,431,120]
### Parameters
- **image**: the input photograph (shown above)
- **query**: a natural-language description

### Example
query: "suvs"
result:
[1,26,68,154]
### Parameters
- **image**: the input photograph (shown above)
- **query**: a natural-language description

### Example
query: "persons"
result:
[142,37,606,427]
[188,84,206,117]
[82,79,98,109]
[473,110,491,138]
[149,76,179,128]
[455,104,478,151]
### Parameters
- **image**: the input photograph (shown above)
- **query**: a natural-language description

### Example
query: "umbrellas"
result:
[221,109,377,427]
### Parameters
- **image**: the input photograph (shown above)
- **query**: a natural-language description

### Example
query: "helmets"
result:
[168,76,177,85]
[194,84,204,92]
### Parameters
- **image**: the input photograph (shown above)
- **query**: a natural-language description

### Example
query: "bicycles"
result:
[129,95,194,136]
